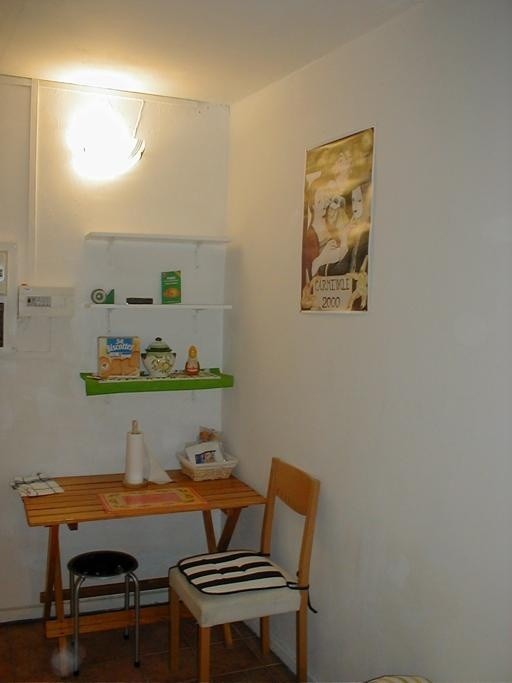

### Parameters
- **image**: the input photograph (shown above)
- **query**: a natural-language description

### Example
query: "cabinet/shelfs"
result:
[82,230,233,389]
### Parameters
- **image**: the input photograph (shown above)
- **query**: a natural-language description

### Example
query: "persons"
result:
[302,139,368,312]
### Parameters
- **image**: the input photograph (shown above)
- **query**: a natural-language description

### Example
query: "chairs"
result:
[167,456,321,681]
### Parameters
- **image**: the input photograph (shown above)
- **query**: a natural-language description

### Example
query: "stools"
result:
[68,549,141,676]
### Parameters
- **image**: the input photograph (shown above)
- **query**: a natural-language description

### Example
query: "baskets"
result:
[176,445,238,481]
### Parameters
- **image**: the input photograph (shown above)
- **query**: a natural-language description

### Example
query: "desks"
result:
[17,467,266,678]
